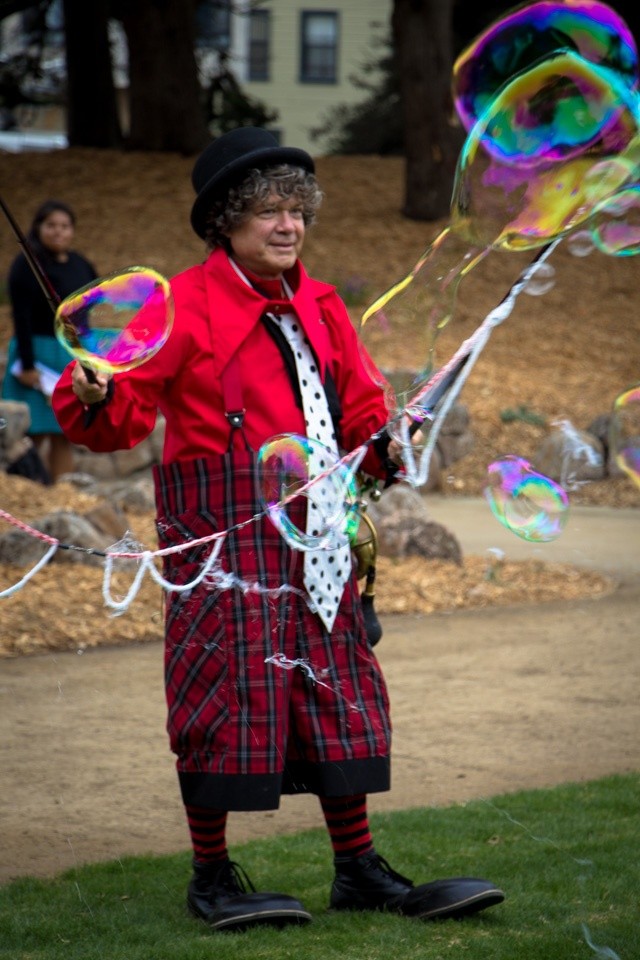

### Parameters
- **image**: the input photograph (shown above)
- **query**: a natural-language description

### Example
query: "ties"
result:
[271,321,356,630]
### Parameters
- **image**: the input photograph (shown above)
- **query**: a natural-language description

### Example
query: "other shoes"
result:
[329,854,505,921]
[182,861,311,928]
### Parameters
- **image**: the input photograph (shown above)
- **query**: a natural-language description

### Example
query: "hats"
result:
[184,131,320,241]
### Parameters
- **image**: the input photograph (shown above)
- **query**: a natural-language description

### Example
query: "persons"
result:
[48,143,424,930]
[6,199,106,496]
